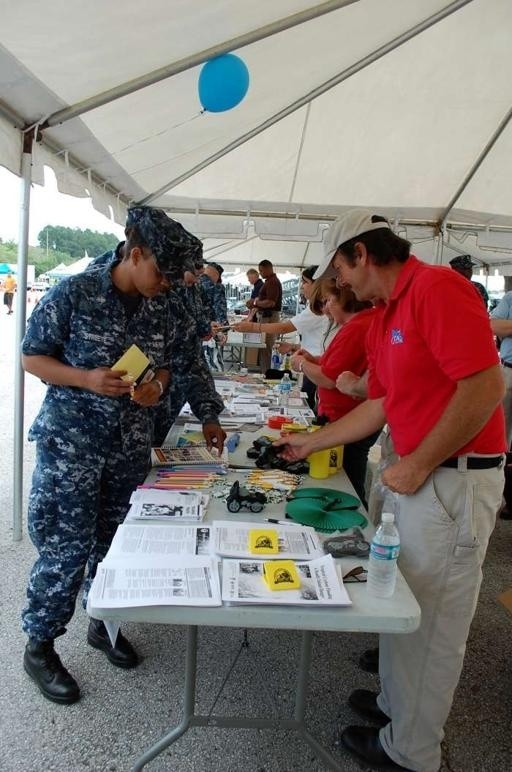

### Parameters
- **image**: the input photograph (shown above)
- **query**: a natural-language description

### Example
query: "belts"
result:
[501,358,512,368]
[438,456,505,469]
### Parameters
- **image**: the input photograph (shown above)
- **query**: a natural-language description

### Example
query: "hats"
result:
[449,255,477,268]
[310,208,391,280]
[128,206,204,274]
[209,263,223,283]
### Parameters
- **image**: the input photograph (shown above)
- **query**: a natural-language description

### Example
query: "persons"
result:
[18,207,228,706]
[83,255,511,534]
[269,209,508,772]
[3,273,16,315]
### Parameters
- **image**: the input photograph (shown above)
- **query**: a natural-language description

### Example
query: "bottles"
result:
[284,353,291,370]
[279,373,291,407]
[365,511,400,599]
[311,412,345,479]
[270,342,282,369]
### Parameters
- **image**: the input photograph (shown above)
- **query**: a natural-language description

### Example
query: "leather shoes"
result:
[88,617,138,668]
[24,638,80,704]
[341,649,418,772]
